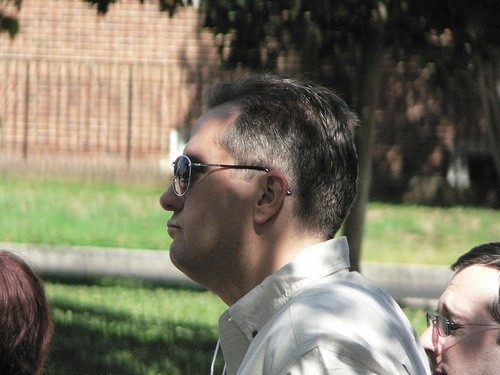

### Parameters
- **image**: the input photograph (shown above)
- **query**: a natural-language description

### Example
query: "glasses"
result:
[426,310,500,335]
[171,155,292,197]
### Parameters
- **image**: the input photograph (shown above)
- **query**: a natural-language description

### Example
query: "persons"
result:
[418,242,500,375]
[160,74,433,375]
